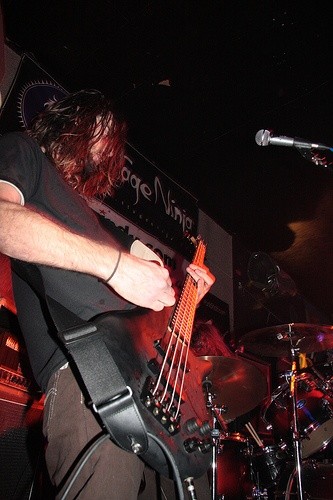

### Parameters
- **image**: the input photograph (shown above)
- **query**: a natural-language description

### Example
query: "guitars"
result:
[85,234,215,480]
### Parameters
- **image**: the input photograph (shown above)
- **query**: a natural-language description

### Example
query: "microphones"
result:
[255,129,329,150]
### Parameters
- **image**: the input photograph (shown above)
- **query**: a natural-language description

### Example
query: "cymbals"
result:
[196,355,266,419]
[238,322,333,354]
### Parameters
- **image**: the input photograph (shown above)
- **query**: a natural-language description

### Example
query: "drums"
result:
[208,434,258,500]
[257,371,333,462]
[252,444,293,494]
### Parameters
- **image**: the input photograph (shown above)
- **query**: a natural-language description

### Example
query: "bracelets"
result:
[104,250,123,282]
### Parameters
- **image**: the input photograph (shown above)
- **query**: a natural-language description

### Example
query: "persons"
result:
[1,86,215,500]
